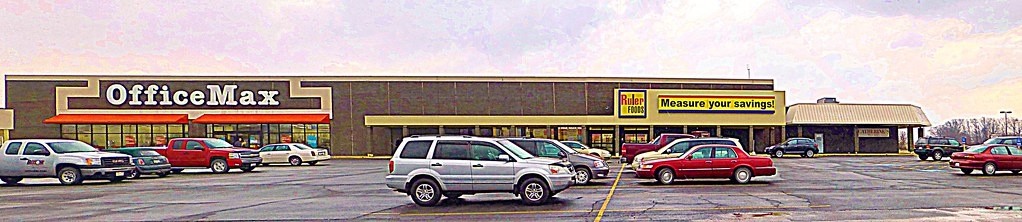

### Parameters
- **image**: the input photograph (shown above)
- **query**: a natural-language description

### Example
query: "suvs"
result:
[631,136,745,172]
[982,135,1022,149]
[0,138,137,186]
[384,133,577,207]
[763,138,820,158]
[487,136,610,187]
[914,135,964,161]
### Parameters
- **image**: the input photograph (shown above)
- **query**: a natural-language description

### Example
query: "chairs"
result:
[702,149,710,158]
[485,147,497,160]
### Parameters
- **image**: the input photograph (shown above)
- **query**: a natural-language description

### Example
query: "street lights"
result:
[1000,110,1012,134]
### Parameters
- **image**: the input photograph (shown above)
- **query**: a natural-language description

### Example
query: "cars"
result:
[635,143,777,186]
[542,139,612,164]
[255,141,332,166]
[948,143,1022,180]
[101,146,171,181]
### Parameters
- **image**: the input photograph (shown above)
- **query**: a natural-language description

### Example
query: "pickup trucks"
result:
[620,132,699,167]
[153,137,262,174]
